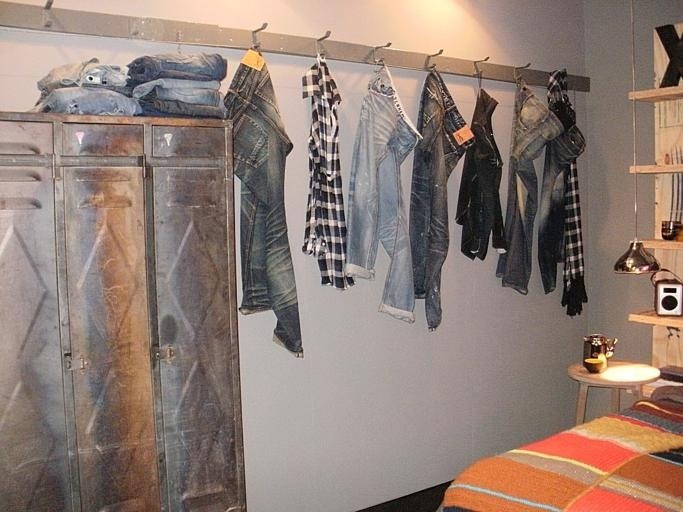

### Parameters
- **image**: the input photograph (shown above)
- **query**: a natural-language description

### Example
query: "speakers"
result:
[654,278,683,317]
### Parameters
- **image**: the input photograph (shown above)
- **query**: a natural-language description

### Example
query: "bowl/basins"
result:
[584,358,604,374]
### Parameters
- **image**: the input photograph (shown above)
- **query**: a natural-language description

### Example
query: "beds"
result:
[434,385,683,512]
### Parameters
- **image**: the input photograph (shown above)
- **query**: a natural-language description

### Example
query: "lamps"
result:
[614,0,661,274]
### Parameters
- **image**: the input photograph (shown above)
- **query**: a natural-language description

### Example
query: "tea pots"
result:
[583,334,618,367]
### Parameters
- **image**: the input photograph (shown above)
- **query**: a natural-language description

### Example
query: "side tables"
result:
[567,358,661,428]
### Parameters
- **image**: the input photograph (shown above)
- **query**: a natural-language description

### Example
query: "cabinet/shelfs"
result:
[0,109,247,512]
[627,85,683,397]
